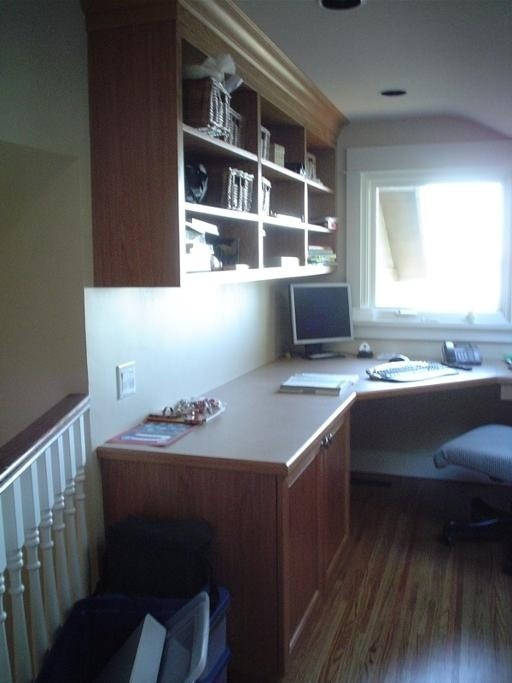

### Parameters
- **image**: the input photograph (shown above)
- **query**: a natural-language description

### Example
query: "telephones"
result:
[442,341,481,365]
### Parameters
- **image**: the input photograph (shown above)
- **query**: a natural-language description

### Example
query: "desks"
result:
[96,351,511,683]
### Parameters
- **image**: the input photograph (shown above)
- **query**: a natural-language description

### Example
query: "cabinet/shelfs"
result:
[86,0,351,287]
[281,418,350,662]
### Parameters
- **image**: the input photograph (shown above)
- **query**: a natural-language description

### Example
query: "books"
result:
[104,415,202,447]
[278,370,361,396]
[146,397,228,424]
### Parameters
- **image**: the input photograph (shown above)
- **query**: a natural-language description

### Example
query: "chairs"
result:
[433,424,511,572]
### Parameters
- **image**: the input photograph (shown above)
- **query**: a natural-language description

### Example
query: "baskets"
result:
[184,77,251,149]
[205,166,255,212]
[260,177,273,216]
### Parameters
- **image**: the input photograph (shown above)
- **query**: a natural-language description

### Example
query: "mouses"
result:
[389,354,410,362]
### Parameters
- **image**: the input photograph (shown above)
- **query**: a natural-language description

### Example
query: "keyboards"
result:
[366,360,459,381]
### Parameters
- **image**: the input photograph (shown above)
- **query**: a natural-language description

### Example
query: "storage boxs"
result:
[96,581,233,683]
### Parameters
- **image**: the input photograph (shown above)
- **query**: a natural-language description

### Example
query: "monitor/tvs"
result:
[289,283,355,360]
